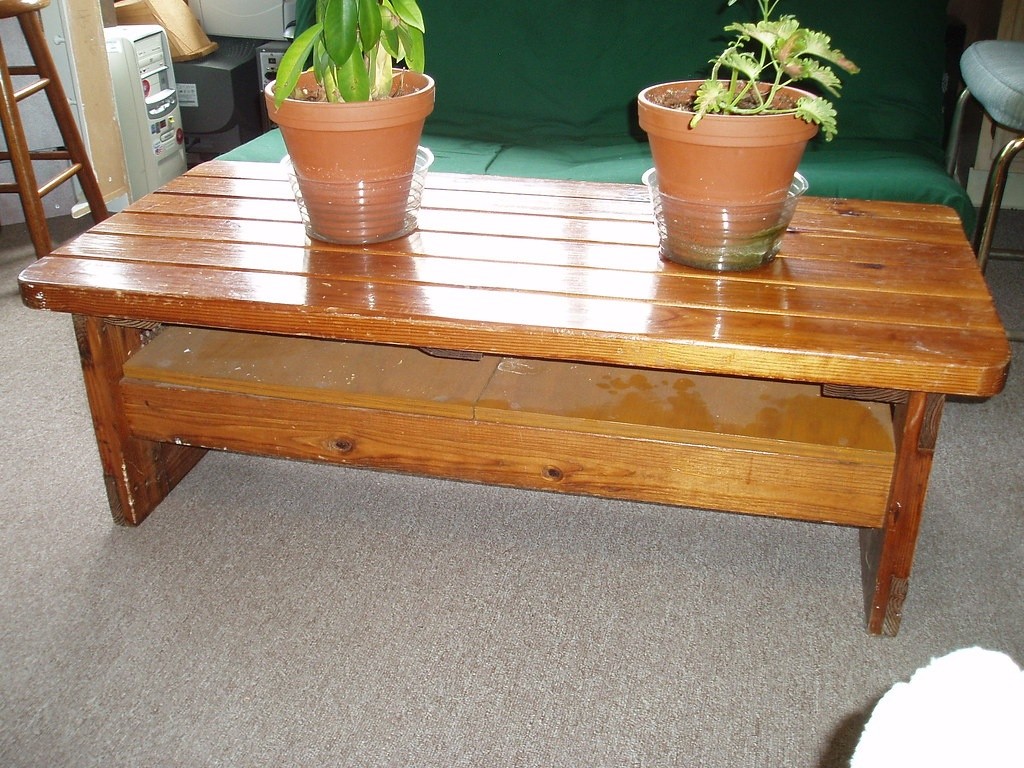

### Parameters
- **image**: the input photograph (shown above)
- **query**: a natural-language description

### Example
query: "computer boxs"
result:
[103,23,189,201]
[256,41,314,133]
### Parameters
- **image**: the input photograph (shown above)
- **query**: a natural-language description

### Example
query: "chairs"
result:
[945,39,1024,279]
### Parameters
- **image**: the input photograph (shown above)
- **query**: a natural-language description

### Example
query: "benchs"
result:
[216,1,977,240]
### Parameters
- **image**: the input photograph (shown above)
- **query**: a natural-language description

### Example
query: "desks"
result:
[14,157,1014,637]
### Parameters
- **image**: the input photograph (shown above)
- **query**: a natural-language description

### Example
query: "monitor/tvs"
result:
[173,34,271,171]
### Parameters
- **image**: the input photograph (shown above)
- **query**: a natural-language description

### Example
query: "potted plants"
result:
[266,0,436,243]
[635,0,861,275]
